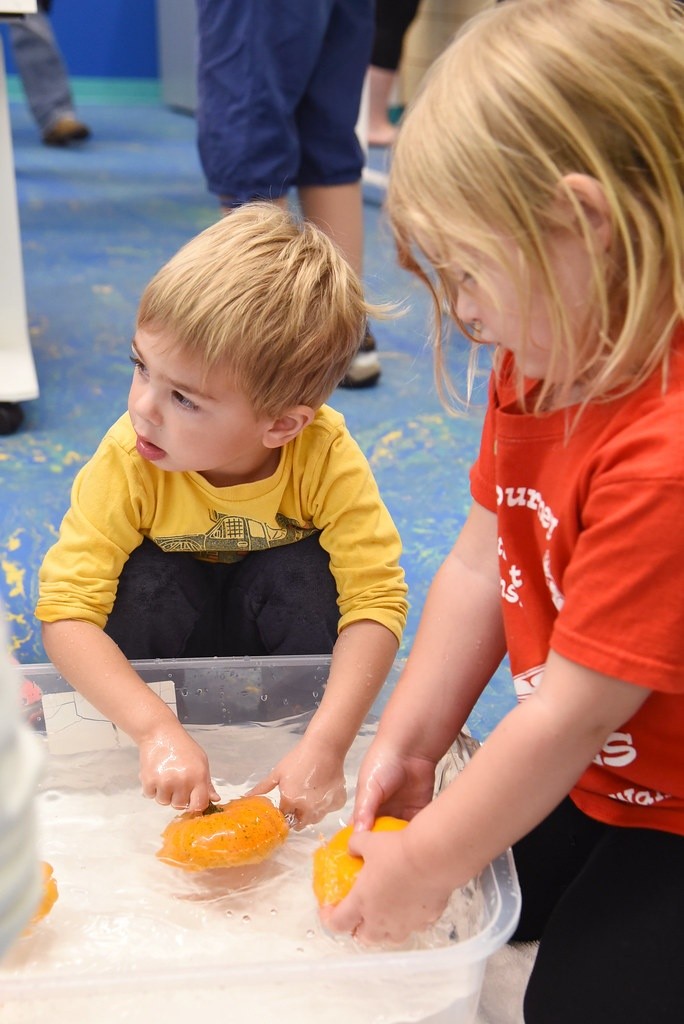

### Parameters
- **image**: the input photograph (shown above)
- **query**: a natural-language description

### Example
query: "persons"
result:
[37,205,408,834]
[11,0,92,147]
[317,0,684,1024]
[367,0,422,146]
[194,0,376,350]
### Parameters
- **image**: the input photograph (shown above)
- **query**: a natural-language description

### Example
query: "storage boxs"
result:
[0,648,524,1024]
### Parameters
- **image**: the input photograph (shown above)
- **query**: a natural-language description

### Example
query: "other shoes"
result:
[38,113,92,146]
[336,329,383,388]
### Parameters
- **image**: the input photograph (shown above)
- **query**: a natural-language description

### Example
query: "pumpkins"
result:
[313,816,411,906]
[157,795,289,871]
[24,861,60,935]
